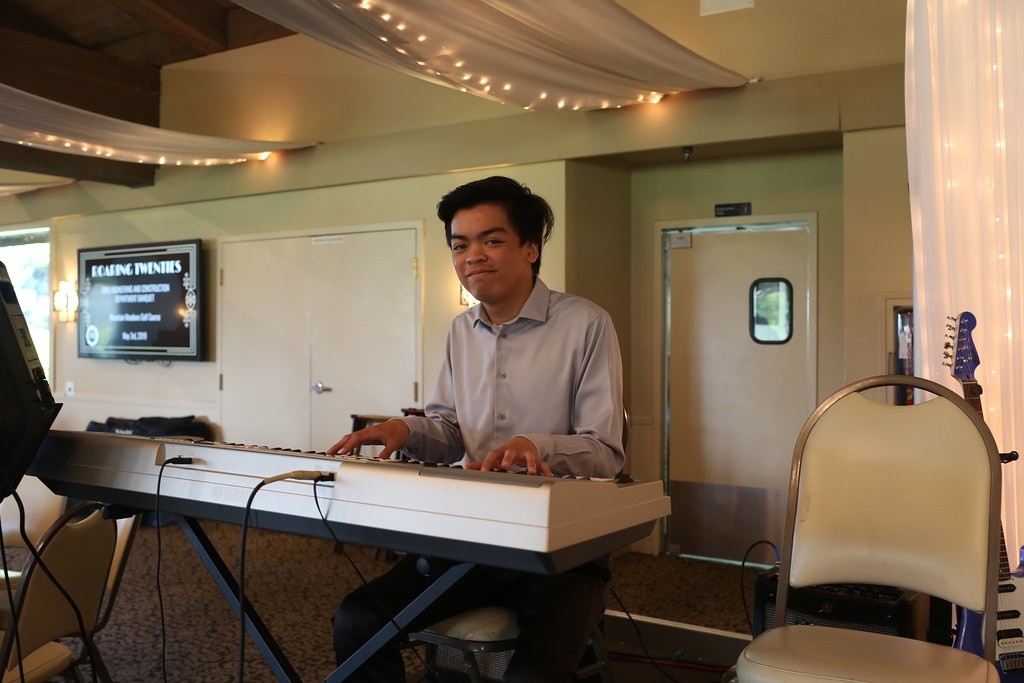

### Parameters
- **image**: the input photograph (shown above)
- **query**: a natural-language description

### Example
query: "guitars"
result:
[943,311,1024,682]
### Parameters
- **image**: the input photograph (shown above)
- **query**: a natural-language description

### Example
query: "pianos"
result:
[31,429,671,683]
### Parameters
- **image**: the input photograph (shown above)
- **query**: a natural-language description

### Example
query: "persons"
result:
[329,175,626,683]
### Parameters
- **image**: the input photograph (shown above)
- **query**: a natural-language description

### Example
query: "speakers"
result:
[752,565,951,648]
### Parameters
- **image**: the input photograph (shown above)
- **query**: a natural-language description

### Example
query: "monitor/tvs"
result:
[76,237,204,361]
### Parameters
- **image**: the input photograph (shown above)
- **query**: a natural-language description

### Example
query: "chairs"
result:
[0,500,119,683]
[715,374,1005,683]
[405,400,635,683]
